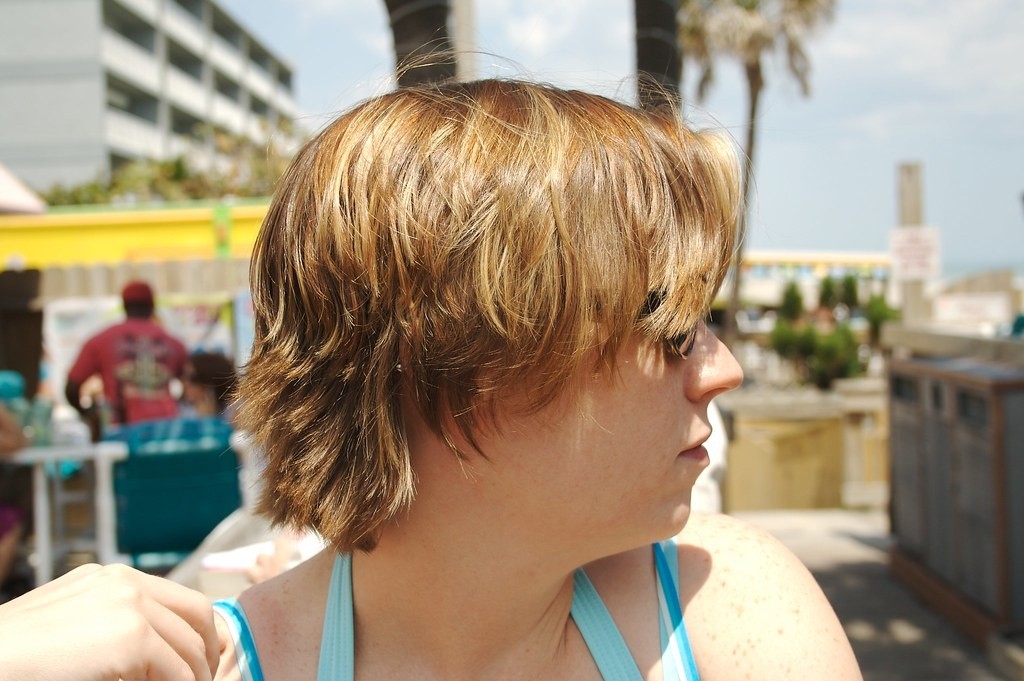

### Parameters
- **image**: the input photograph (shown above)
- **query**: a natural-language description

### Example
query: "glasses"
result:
[567,290,696,357]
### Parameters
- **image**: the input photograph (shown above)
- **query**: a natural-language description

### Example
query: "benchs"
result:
[0,419,257,603]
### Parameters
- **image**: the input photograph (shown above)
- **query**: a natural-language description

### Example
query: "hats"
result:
[122,282,152,302]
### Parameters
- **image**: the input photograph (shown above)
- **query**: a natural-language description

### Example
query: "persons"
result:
[0,402,31,581]
[177,351,240,417]
[65,280,191,427]
[0,78,863,681]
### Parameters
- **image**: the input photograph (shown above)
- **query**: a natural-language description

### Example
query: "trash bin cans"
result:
[884,356,1024,647]
